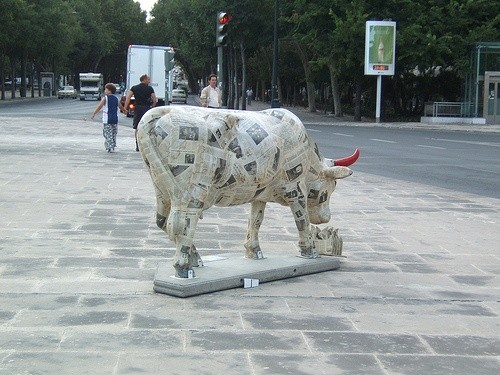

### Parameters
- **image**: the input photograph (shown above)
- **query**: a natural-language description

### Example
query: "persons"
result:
[199,74,222,108]
[246,88,253,105]
[92,84,128,152]
[124,74,156,151]
[179,128,198,140]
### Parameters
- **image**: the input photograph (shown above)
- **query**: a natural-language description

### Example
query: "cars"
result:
[113,81,125,94]
[172,68,188,104]
[57,86,77,99]
[120,90,126,112]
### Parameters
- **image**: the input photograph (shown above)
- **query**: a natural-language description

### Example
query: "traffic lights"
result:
[216,12,232,47]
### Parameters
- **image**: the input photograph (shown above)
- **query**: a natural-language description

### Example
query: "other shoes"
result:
[107,148,115,152]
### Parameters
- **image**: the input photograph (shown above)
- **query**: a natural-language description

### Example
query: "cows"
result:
[134,104,360,278]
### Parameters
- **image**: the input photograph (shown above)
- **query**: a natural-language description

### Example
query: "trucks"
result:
[79,73,103,101]
[125,45,175,117]
